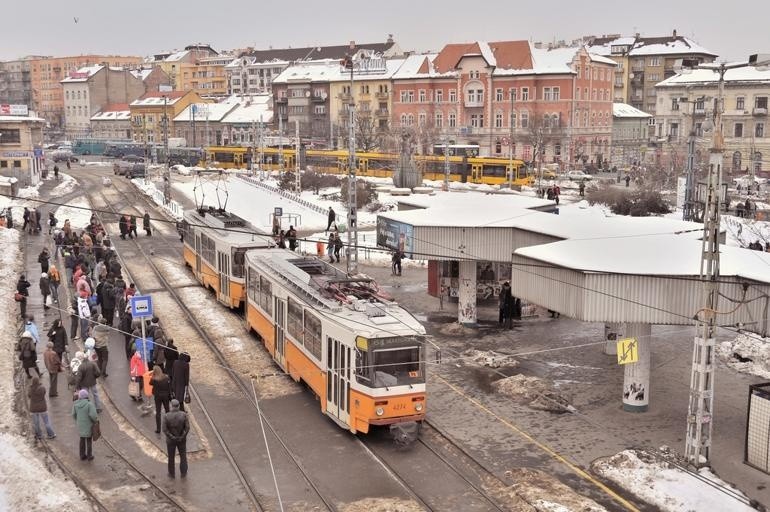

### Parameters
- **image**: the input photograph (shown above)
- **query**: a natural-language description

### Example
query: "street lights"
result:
[663,49,769,485]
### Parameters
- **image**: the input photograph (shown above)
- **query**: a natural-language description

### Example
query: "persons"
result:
[748,240,770,252]
[347,210,354,227]
[325,207,337,231]
[67,159,71,168]
[284,226,296,251]
[625,174,630,187]
[3,207,191,479]
[326,233,341,262]
[391,253,402,277]
[579,181,585,196]
[499,282,514,330]
[279,230,286,249]
[54,165,59,180]
[737,198,756,219]
[536,184,560,205]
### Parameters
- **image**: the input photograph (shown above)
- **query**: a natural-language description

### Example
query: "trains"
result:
[192,136,539,193]
[172,172,433,439]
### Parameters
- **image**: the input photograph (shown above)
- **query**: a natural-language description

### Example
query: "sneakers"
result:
[81,454,95,462]
[35,432,57,439]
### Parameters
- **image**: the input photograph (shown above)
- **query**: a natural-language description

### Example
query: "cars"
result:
[43,137,151,182]
[530,159,647,184]
[730,172,769,192]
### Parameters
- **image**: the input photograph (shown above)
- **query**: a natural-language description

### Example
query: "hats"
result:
[22,330,32,338]
[78,389,89,399]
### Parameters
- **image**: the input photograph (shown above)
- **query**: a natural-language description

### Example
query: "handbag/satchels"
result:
[185,385,191,403]
[67,305,76,316]
[18,350,25,360]
[14,292,24,302]
[127,378,141,398]
[90,419,102,442]
[48,325,56,341]
[45,293,53,306]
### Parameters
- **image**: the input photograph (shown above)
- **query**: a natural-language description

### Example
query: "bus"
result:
[151,144,206,169]
